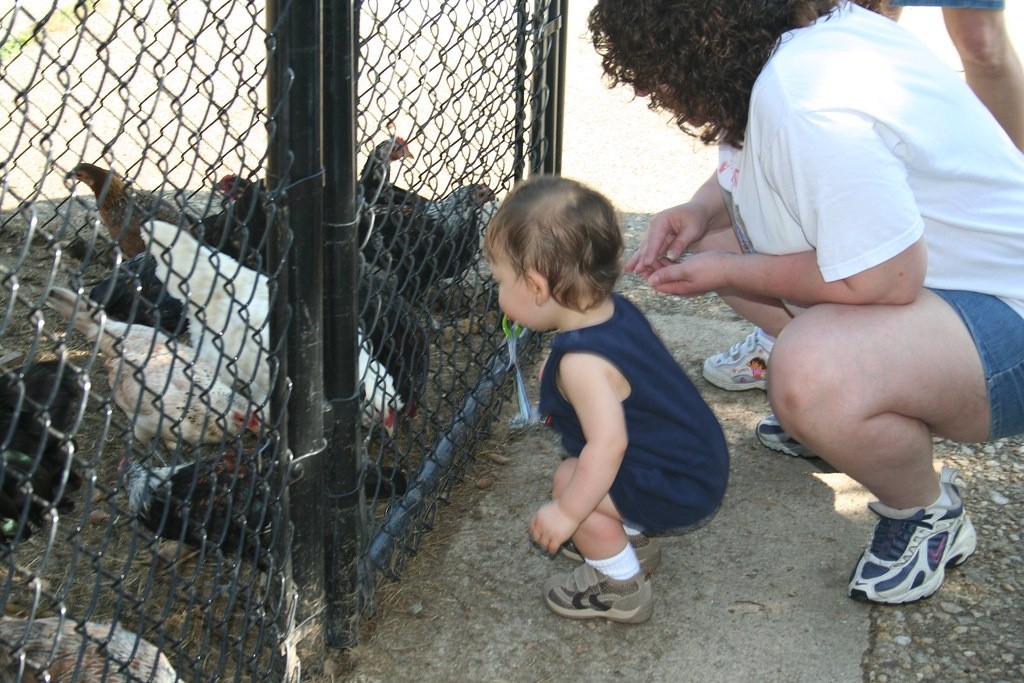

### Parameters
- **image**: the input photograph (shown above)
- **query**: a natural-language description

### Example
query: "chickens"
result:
[0,135,501,683]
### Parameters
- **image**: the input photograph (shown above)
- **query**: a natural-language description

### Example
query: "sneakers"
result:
[542,562,654,623]
[558,532,662,573]
[848,467,977,604]
[702,328,778,391]
[756,412,818,457]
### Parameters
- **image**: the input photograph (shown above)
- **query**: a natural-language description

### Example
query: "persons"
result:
[583,0,1024,607]
[482,177,731,625]
